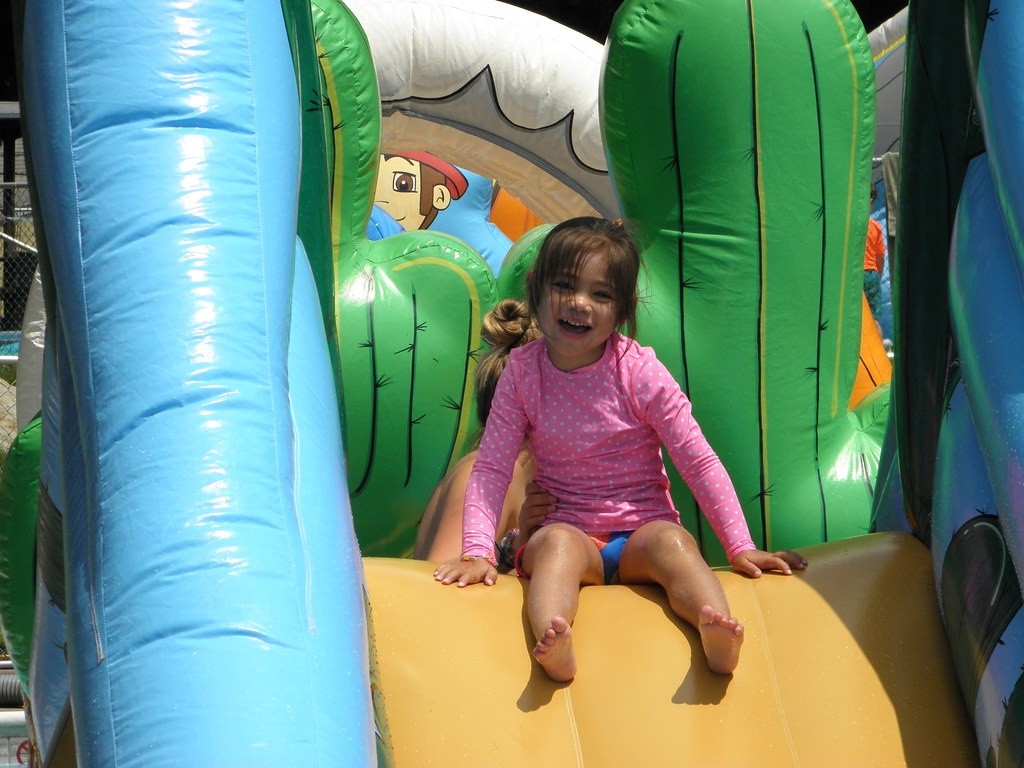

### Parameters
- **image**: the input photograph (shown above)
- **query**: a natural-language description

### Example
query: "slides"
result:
[0,0,1024,764]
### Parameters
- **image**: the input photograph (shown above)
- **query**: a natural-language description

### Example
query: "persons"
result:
[415,299,557,578]
[433,217,806,684]
[863,184,886,340]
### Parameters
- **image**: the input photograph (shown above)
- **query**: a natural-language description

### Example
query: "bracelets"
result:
[462,554,499,569]
[500,528,519,568]
